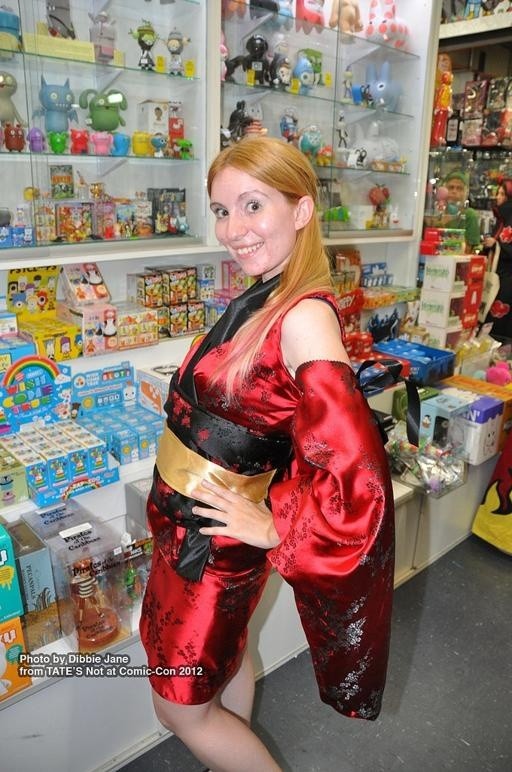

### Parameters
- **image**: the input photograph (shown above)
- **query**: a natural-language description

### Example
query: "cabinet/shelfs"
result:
[1,340,503,771]
[1,2,218,446]
[209,0,431,349]
[414,0,512,391]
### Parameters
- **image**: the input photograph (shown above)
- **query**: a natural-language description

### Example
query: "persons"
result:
[431,70,454,146]
[484,177,512,361]
[433,171,480,254]
[143,134,393,769]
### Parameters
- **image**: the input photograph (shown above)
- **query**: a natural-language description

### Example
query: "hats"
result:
[447,170,469,186]
